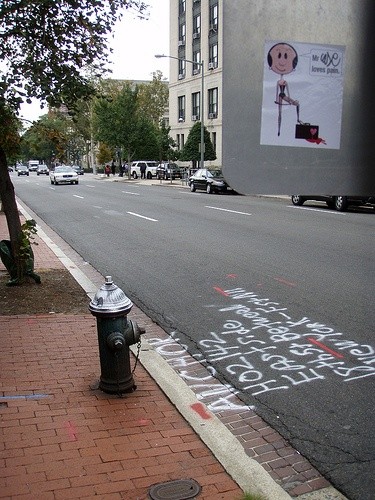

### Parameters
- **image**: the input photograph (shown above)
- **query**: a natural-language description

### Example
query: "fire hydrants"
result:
[88,275,147,396]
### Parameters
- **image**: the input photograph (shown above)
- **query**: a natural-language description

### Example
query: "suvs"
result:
[130,160,161,179]
[156,163,185,180]
[187,168,236,194]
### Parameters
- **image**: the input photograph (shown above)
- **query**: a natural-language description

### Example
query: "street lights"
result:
[154,53,205,169]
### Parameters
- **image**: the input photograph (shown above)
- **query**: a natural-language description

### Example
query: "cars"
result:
[72,165,85,175]
[8,160,40,176]
[290,194,375,214]
[36,164,50,175]
[49,166,80,185]
